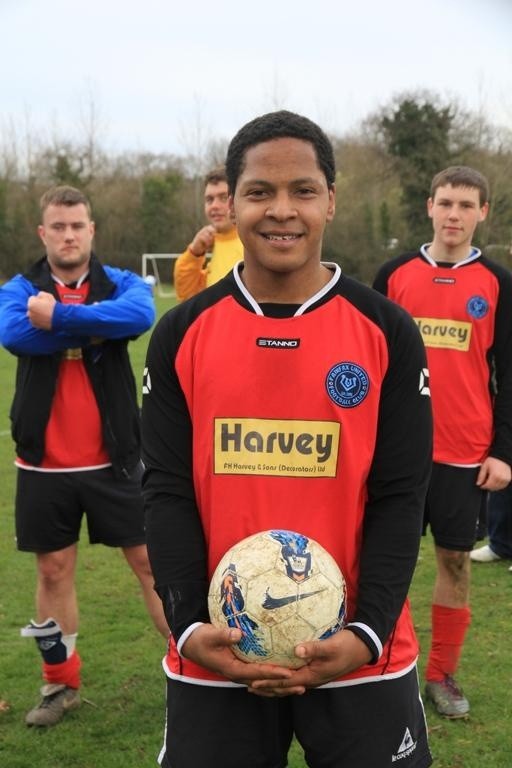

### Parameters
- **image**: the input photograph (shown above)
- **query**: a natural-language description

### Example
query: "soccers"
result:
[207,530,347,670]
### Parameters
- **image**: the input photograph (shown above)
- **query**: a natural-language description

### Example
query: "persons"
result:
[469,244,512,570]
[136,108,435,768]
[369,164,512,723]
[1,184,180,728]
[173,161,246,305]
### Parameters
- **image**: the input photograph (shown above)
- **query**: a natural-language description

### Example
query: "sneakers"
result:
[424,679,468,719]
[470,544,501,563]
[25,686,81,727]
